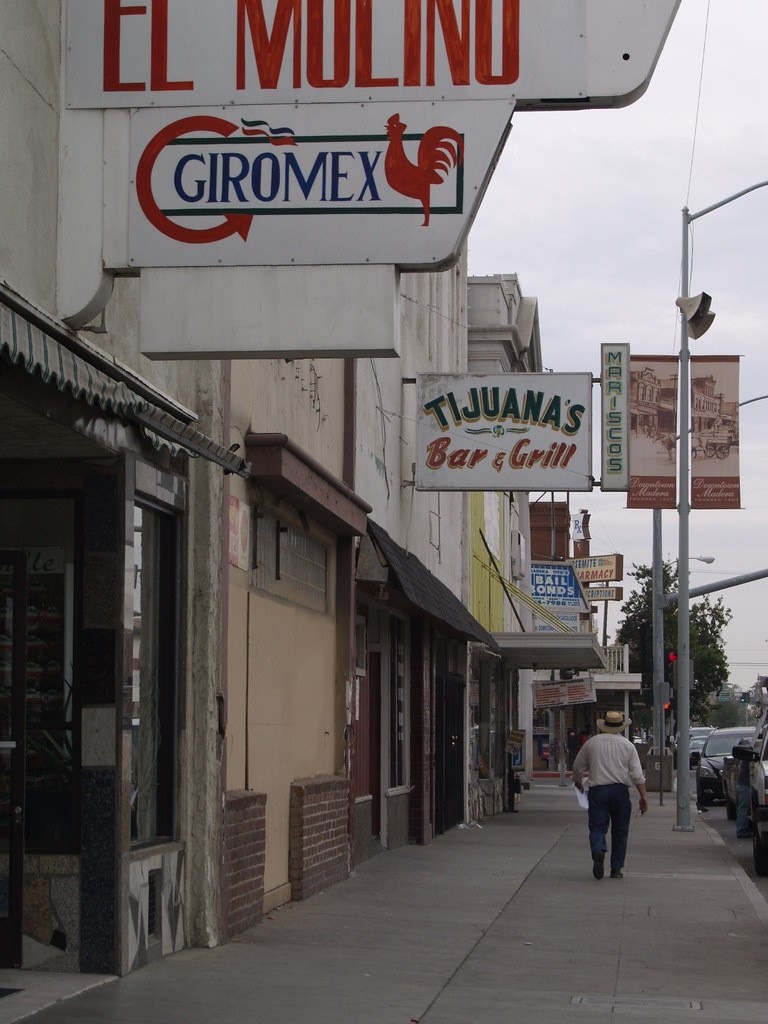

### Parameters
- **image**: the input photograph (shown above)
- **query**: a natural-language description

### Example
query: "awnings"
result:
[373,523,501,653]
[0,304,251,479]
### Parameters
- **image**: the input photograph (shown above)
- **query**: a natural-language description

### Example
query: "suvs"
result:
[691,726,763,808]
[732,723,768,878]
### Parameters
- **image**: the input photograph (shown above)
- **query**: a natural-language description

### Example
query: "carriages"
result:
[647,423,734,462]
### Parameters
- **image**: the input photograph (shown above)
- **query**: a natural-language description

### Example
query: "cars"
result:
[720,735,753,821]
[672,723,718,770]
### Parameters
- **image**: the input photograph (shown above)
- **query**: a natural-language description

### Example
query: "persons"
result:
[567,731,593,771]
[572,711,648,879]
[735,740,753,838]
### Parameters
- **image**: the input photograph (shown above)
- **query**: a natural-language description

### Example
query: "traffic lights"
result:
[663,696,678,710]
[640,622,678,678]
[740,692,750,704]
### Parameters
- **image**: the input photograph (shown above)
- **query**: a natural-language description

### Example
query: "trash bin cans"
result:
[645,747,674,792]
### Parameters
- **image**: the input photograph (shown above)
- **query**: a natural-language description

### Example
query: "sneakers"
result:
[593,851,605,879]
[611,868,623,878]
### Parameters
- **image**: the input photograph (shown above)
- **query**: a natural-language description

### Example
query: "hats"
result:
[596,711,629,734]
